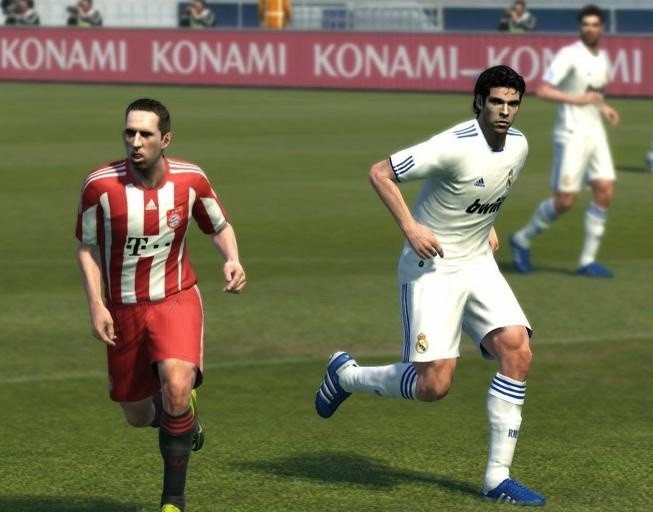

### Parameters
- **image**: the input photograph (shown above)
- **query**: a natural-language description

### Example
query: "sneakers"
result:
[313,348,360,420]
[481,476,548,508]
[505,234,616,282]
